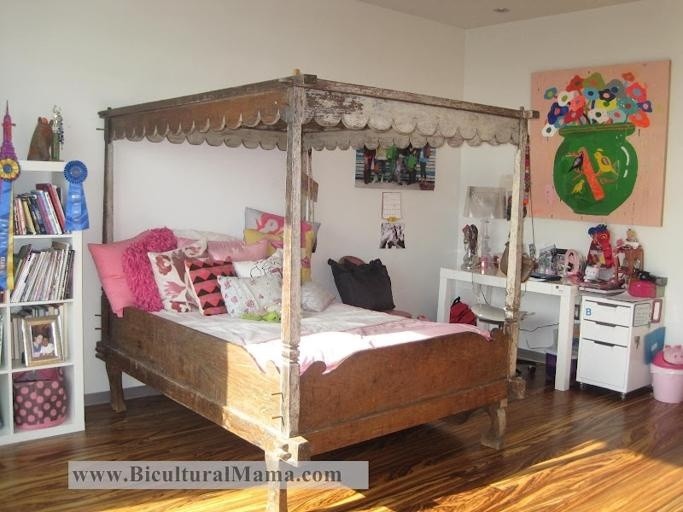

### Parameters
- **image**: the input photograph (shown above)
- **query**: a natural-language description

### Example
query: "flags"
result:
[663,345,683,365]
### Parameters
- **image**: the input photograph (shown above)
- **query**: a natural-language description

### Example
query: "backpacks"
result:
[449,296,477,324]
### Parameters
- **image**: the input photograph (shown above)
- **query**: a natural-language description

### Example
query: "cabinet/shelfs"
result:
[0,157,87,444]
[577,295,655,394]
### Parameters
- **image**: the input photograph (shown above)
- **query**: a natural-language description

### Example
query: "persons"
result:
[363,144,433,185]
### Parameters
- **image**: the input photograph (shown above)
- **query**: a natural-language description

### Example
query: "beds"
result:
[99,67,541,511]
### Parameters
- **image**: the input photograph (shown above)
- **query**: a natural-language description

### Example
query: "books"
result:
[11,184,76,360]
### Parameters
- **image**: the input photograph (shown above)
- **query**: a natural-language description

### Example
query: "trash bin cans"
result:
[649,344,683,404]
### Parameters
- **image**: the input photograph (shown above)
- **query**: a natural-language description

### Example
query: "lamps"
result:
[464,185,507,274]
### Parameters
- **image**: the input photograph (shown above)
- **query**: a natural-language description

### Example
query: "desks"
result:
[436,267,584,392]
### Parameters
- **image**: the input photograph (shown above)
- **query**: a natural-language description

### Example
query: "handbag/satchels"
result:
[326,254,393,311]
[500,244,535,283]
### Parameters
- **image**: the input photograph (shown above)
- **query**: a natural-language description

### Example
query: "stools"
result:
[470,302,537,380]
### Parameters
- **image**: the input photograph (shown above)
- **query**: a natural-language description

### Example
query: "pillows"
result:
[87,207,337,319]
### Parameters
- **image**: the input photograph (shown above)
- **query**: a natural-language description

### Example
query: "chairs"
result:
[328,255,411,319]
